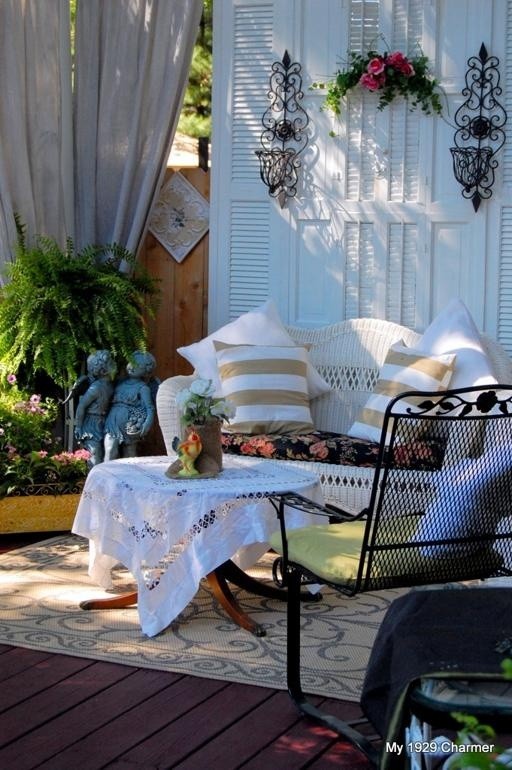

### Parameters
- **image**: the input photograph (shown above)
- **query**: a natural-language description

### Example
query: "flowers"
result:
[0,373,92,499]
[173,374,238,427]
[306,35,452,137]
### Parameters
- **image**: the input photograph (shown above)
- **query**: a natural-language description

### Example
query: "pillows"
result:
[175,297,333,401]
[346,336,456,449]
[211,339,323,437]
[414,294,500,417]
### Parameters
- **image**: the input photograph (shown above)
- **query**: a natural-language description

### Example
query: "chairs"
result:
[265,380,512,770]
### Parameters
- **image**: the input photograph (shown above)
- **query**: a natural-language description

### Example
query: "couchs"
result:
[155,316,512,522]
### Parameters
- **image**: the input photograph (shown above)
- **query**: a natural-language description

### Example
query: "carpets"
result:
[0,535,485,704]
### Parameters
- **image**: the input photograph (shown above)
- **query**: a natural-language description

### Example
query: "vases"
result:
[178,421,223,472]
[0,482,89,534]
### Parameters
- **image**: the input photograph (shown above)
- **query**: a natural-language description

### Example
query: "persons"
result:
[74,349,157,475]
